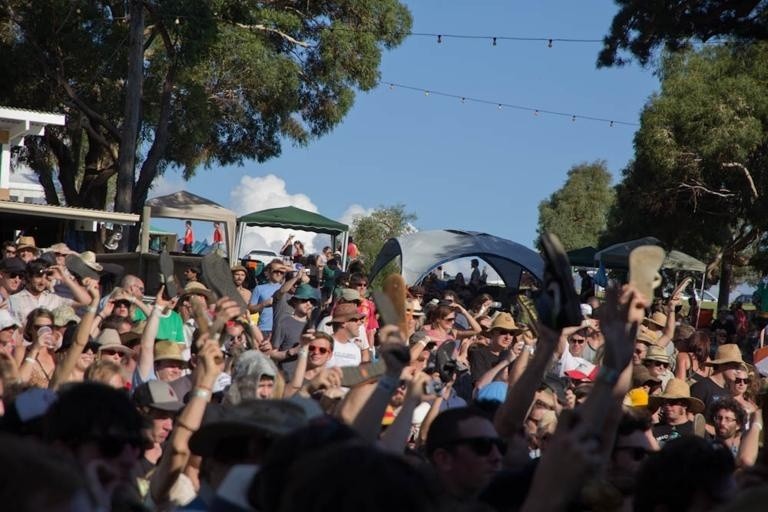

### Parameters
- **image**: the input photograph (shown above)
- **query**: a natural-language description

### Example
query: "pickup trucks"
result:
[243,249,283,267]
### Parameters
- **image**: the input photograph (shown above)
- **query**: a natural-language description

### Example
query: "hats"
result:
[704,343,746,369]
[0,235,705,414]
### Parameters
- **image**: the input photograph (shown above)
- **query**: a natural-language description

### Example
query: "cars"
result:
[662,286,718,302]
[731,294,754,304]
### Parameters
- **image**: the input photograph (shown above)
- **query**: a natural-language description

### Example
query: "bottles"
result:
[490,302,501,308]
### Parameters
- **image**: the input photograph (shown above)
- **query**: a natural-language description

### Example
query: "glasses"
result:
[712,415,739,423]
[734,376,751,385]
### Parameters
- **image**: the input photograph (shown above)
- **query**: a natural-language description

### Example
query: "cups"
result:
[37,326,53,346]
